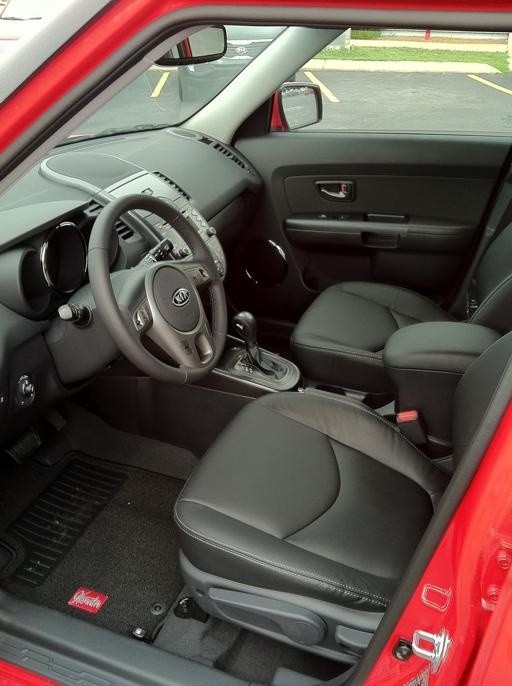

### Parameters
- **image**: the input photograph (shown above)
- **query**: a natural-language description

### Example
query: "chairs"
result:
[173,332,511,666]
[291,221,512,394]
